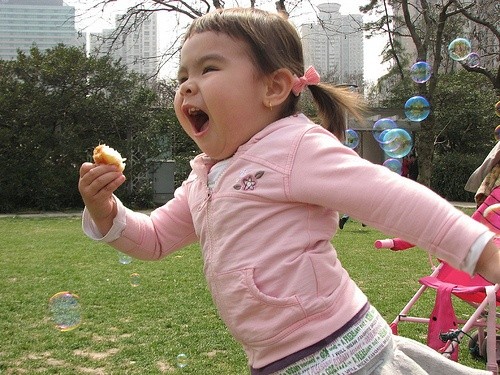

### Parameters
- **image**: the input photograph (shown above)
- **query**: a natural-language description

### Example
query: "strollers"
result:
[374,178,500,373]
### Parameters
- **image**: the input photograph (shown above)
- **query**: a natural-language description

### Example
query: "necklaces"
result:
[78,8,499,375]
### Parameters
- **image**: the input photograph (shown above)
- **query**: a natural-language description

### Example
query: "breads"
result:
[92,144,126,173]
